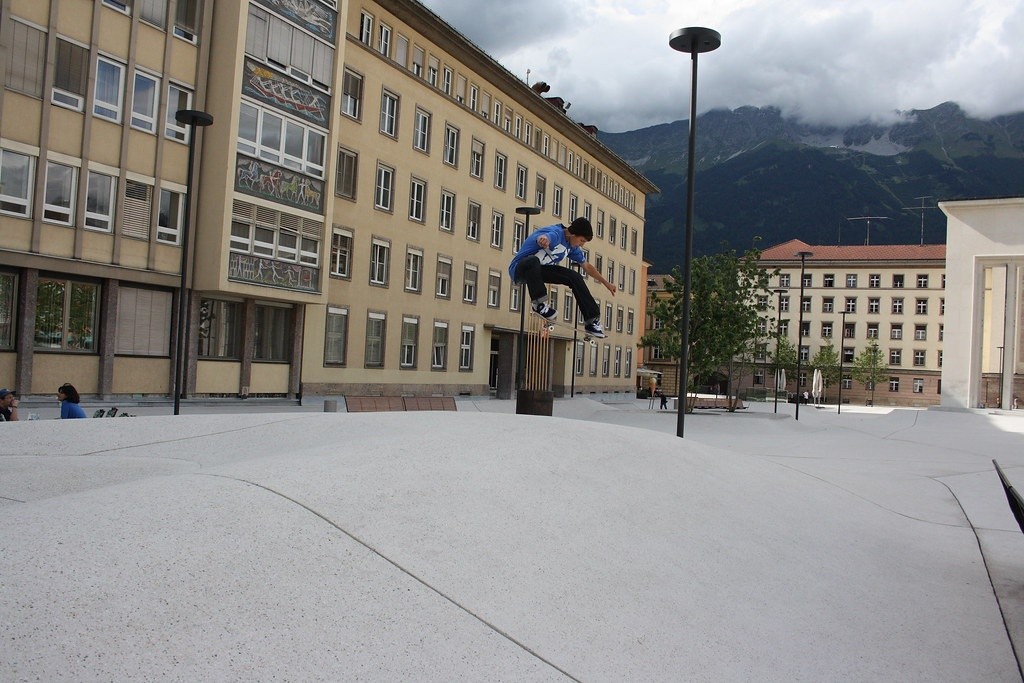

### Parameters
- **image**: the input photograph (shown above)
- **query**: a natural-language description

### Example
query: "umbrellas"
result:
[703,370,730,388]
[773,368,780,391]
[637,368,663,388]
[817,369,824,404]
[780,369,787,390]
[812,369,818,403]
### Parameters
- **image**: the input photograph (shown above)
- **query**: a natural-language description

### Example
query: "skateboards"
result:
[530,311,609,346]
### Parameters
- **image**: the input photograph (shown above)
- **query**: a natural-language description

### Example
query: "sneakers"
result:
[585,319,605,338]
[532,303,558,320]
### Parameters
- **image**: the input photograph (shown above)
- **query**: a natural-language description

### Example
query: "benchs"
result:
[686,397,744,408]
[342,393,405,412]
[403,396,458,412]
[993,458,1024,534]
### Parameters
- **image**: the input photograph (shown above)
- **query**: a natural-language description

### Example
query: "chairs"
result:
[93,407,129,418]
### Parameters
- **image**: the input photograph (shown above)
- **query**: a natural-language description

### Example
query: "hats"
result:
[0,389,16,398]
[572,217,593,237]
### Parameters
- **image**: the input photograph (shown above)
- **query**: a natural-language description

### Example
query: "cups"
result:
[28,412,40,420]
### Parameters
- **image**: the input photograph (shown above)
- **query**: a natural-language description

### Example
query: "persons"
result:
[0,388,21,422]
[57,383,88,419]
[803,389,809,405]
[508,217,616,338]
[638,386,660,396]
[1013,397,1019,409]
[68,329,74,349]
[660,392,668,410]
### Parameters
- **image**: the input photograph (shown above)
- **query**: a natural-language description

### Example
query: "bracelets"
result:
[12,406,17,408]
